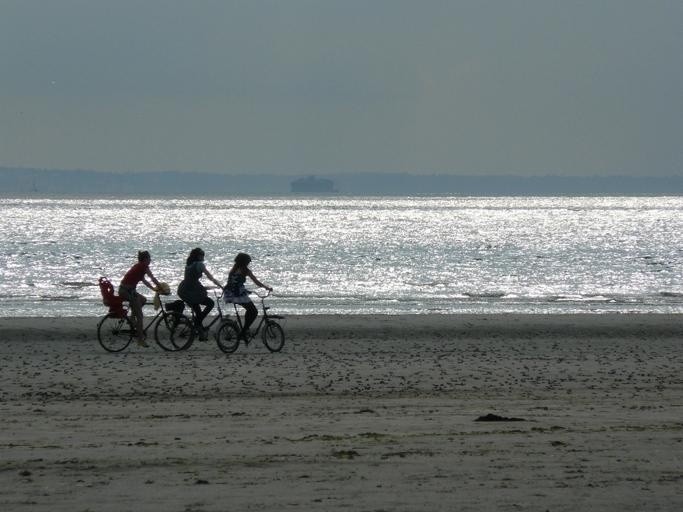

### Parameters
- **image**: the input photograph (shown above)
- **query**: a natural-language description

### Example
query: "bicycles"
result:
[216,288,285,353]
[96,290,193,353]
[154,291,239,354]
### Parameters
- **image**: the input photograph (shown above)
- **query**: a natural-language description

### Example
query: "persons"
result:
[221,253,273,337]
[176,247,221,343]
[117,249,161,348]
